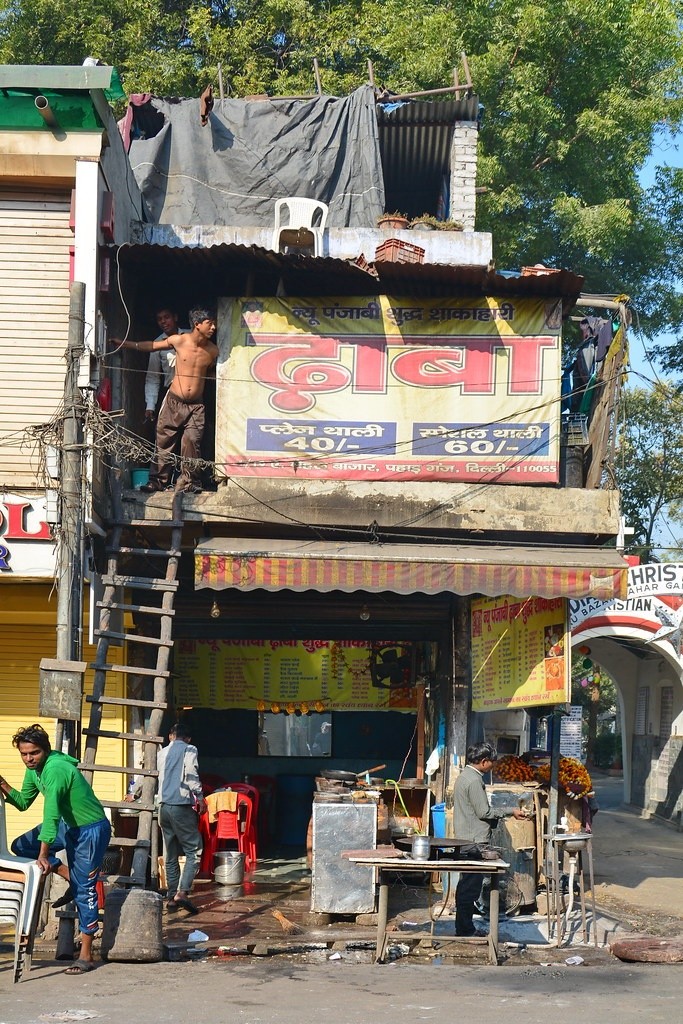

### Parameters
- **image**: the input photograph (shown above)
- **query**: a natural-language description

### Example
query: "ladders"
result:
[56,490,187,963]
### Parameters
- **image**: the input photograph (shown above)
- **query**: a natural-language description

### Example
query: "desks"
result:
[354,862,505,966]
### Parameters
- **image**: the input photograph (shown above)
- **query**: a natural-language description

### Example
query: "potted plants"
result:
[410,213,439,230]
[376,209,410,229]
[436,217,467,231]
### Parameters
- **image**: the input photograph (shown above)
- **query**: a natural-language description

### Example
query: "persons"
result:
[111,306,219,495]
[0,724,111,976]
[124,722,208,914]
[453,741,526,939]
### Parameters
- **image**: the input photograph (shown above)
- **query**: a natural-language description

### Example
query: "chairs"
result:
[199,791,254,874]
[272,196,329,258]
[0,792,46,984]
[219,782,259,863]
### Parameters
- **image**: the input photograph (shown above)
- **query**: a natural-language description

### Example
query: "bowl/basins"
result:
[404,829,414,834]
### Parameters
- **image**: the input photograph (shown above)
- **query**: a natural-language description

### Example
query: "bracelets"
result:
[0,780,5,785]
[136,342,139,351]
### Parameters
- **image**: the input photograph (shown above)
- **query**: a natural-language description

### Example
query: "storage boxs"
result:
[374,238,425,264]
[561,412,590,446]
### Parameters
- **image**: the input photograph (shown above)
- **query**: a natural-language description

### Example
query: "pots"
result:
[320,764,386,781]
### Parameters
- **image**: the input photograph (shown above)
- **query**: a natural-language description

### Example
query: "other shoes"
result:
[184,483,203,493]
[454,930,485,936]
[140,480,163,492]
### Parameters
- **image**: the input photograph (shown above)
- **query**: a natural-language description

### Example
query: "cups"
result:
[411,836,430,861]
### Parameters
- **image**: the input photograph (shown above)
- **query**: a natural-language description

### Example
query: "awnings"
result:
[194,535,629,600]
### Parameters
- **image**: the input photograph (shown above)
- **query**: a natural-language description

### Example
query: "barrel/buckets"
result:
[210,851,245,885]
[431,803,446,850]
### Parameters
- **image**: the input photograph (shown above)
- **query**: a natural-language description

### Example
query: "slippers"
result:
[52,896,73,908]
[167,903,178,913]
[64,959,96,975]
[175,898,199,914]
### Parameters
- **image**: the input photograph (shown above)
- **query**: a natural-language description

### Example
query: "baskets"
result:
[520,265,561,277]
[374,238,426,264]
